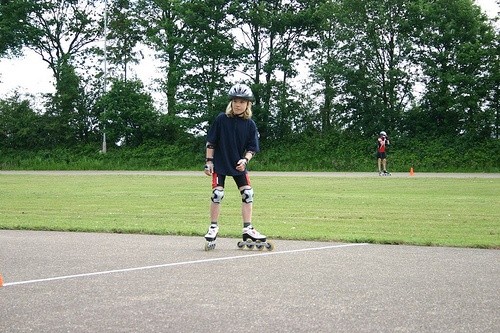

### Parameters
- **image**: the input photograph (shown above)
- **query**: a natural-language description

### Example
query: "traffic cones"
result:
[409,167,415,177]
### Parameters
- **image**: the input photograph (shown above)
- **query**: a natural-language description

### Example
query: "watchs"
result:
[240,155,249,164]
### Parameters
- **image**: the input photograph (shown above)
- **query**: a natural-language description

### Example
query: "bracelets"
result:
[205,158,213,162]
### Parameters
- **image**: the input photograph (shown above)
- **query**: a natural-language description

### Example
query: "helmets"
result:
[380,131,387,137]
[227,84,253,101]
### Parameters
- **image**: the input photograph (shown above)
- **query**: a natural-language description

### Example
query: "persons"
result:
[378,131,390,174]
[204,84,259,242]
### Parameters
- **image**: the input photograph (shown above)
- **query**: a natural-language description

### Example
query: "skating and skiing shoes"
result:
[205,225,219,250]
[237,226,272,251]
[379,170,391,176]
